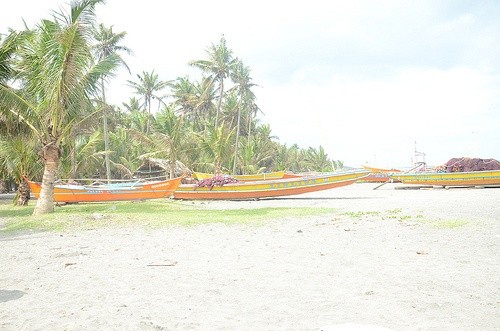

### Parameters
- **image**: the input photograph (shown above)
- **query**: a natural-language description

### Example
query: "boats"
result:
[22,158,500,202]
